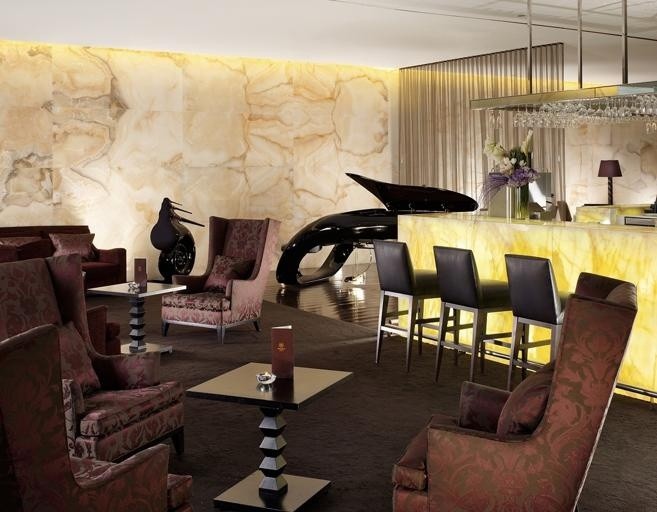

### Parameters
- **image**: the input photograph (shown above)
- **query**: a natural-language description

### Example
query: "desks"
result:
[574,202,653,225]
[181,358,355,512]
[394,211,657,408]
[84,278,186,358]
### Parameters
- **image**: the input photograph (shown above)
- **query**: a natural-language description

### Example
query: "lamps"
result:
[595,159,623,206]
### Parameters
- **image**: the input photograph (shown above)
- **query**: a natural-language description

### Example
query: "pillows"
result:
[491,355,558,440]
[47,319,104,395]
[0,232,42,245]
[200,253,255,295]
[48,227,98,262]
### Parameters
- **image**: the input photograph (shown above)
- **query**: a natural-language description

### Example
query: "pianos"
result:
[276,172,480,288]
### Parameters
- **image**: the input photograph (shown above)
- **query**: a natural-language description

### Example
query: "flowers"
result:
[476,127,542,217]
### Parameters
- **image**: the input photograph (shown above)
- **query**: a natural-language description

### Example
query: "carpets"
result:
[74,297,656,512]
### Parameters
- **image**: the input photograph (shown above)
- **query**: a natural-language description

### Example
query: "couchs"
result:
[0,223,128,297]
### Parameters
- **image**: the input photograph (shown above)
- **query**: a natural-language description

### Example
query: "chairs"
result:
[0,252,189,461]
[388,269,638,511]
[156,215,285,349]
[367,233,465,377]
[426,244,530,383]
[556,200,573,223]
[500,248,572,391]
[0,315,198,512]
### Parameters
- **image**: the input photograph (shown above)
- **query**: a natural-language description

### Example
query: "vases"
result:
[513,185,528,220]
